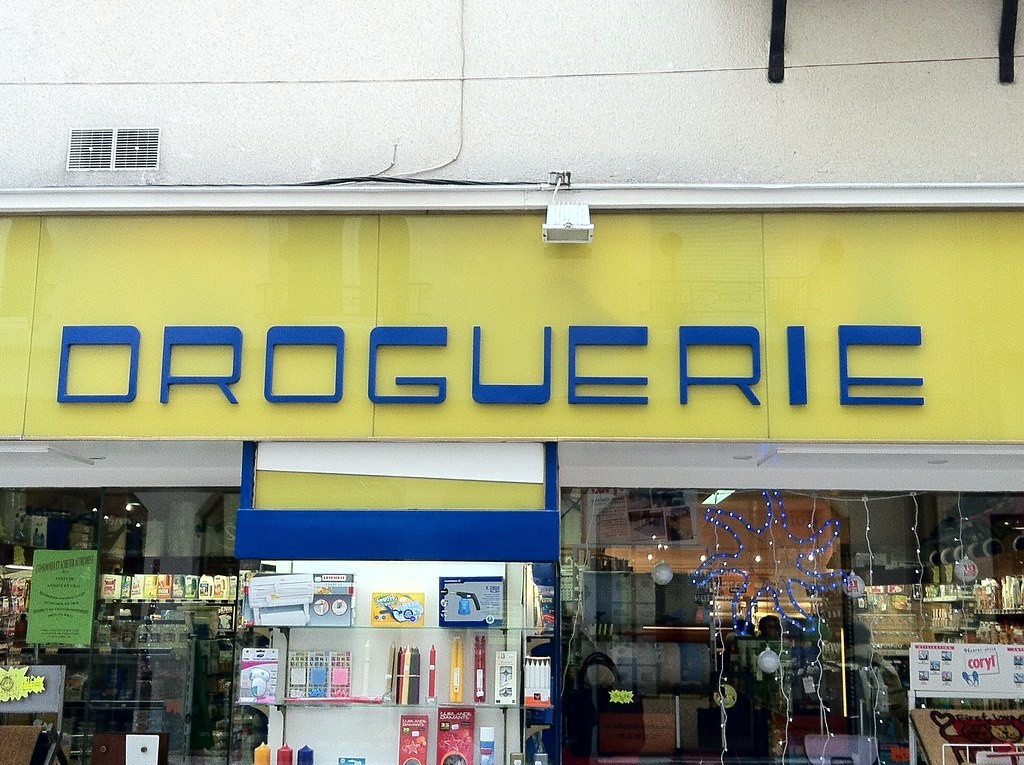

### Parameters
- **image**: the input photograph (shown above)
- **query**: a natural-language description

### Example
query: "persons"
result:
[723,616,907,717]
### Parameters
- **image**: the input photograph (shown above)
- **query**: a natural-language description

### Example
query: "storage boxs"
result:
[664,573,709,620]
[438,576,504,628]
[583,571,612,626]
[607,641,633,666]
[635,573,664,603]
[242,572,356,626]
[611,572,636,604]
[681,643,704,687]
[659,642,681,689]
[637,602,662,630]
[633,642,660,667]
[637,666,658,693]
[239,648,285,703]
[612,604,635,631]
[436,707,475,765]
[616,665,635,692]
[495,651,518,705]
[600,713,676,756]
[398,714,428,765]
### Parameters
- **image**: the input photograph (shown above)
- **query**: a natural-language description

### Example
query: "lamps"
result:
[757,646,779,674]
[841,569,866,598]
[955,553,979,581]
[651,561,673,585]
[541,204,595,244]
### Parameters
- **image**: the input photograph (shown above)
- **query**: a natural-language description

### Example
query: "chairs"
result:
[0,665,70,765]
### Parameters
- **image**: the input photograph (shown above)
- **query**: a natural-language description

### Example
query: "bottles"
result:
[157,573,229,600]
[94,609,187,648]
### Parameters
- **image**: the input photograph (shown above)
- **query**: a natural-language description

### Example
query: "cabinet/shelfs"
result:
[845,553,1024,738]
[226,509,563,765]
[0,610,202,765]
[0,572,247,759]
